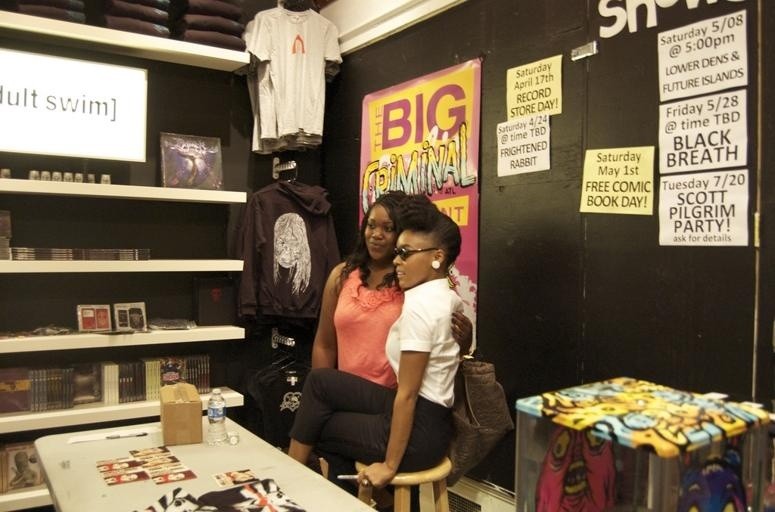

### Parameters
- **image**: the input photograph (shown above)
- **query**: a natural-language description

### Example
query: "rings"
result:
[362,480,368,486]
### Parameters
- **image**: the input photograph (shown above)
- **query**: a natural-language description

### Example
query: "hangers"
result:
[268,0,320,18]
[267,334,310,378]
[274,160,314,192]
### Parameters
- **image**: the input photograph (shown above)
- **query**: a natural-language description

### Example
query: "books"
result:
[2,442,43,496]
[0,354,211,415]
[76,301,199,334]
[0,210,150,260]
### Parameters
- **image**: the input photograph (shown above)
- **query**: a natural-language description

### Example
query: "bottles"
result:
[206,388,225,446]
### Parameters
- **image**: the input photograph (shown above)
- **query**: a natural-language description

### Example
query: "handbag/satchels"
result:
[443,359,513,487]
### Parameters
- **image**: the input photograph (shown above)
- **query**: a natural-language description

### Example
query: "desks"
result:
[32,420,376,512]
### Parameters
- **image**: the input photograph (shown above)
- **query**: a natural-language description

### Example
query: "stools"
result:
[352,457,454,512]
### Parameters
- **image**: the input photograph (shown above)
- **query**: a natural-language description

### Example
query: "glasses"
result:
[393,247,440,259]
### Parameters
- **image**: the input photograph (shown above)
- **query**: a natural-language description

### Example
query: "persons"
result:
[310,191,474,478]
[286,195,464,491]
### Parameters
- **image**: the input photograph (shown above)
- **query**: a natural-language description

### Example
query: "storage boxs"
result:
[158,382,203,447]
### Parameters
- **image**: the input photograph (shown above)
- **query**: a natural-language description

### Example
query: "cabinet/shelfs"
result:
[0,11,252,512]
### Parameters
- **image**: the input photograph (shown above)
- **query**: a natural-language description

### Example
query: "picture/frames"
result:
[76,304,112,332]
[160,131,225,190]
[113,301,148,333]
